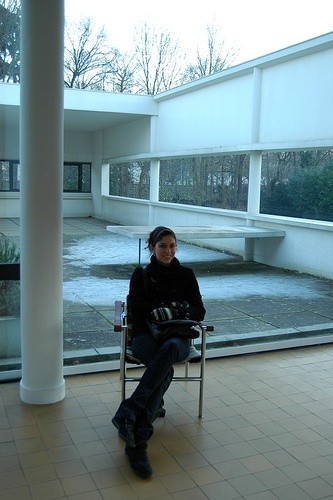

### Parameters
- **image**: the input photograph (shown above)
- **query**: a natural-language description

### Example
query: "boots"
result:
[111,398,153,440]
[124,426,153,479]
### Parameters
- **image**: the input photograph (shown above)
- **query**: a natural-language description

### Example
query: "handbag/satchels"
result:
[161,321,199,339]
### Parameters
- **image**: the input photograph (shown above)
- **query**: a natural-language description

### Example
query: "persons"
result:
[112,227,207,480]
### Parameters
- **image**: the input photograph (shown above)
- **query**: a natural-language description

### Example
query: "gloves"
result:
[150,308,172,321]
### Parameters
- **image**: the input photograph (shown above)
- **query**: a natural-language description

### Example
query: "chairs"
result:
[114,292,214,418]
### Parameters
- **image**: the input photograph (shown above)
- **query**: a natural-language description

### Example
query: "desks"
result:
[107,225,286,267]
